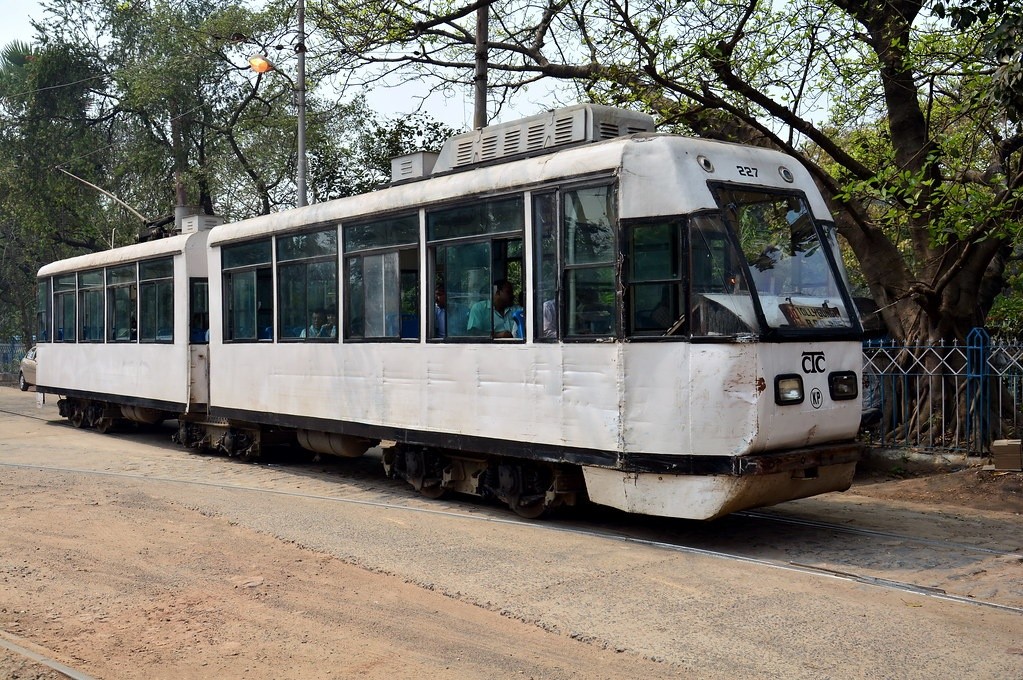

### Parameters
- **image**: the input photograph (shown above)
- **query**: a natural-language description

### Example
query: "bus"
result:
[35,105,865,520]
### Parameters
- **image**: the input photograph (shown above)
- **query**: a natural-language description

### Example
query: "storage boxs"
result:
[992,438,1022,469]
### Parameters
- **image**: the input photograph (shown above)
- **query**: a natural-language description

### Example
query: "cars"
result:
[19,346,40,392]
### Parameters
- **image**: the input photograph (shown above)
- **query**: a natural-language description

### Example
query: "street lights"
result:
[249,55,305,208]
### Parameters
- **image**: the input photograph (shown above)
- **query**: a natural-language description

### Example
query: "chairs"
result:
[41,309,525,341]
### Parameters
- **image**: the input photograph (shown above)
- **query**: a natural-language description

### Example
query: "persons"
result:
[650,283,684,331]
[300,307,339,339]
[432,270,599,340]
[116,315,138,342]
